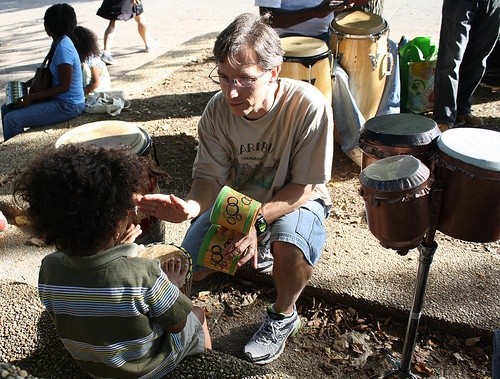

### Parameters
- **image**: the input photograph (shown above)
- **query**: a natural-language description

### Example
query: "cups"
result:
[196,224,249,276]
[209,185,262,235]
[6,81,26,105]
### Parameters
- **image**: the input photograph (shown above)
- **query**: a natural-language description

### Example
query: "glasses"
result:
[209,64,268,87]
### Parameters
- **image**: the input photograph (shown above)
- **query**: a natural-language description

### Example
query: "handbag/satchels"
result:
[83,92,131,117]
[29,67,52,102]
[397,45,438,114]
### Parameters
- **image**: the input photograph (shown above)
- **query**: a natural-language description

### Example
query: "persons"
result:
[133,12,334,366]
[432,0,500,132]
[255,0,402,167]
[1,3,86,141]
[11,143,213,379]
[96,0,151,65]
[68,26,112,99]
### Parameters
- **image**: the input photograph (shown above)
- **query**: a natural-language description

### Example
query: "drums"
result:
[142,241,194,296]
[278,36,334,108]
[53,118,166,240]
[434,126,499,243]
[195,187,262,275]
[326,9,392,126]
[357,113,437,166]
[359,153,434,252]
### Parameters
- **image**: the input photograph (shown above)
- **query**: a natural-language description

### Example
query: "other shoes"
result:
[454,114,485,129]
[436,123,454,134]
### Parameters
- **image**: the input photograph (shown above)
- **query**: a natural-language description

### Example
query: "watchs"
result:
[255,213,268,238]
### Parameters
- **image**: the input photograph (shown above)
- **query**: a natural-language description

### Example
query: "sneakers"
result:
[143,38,158,51]
[243,301,301,363]
[100,54,115,64]
[251,228,274,273]
